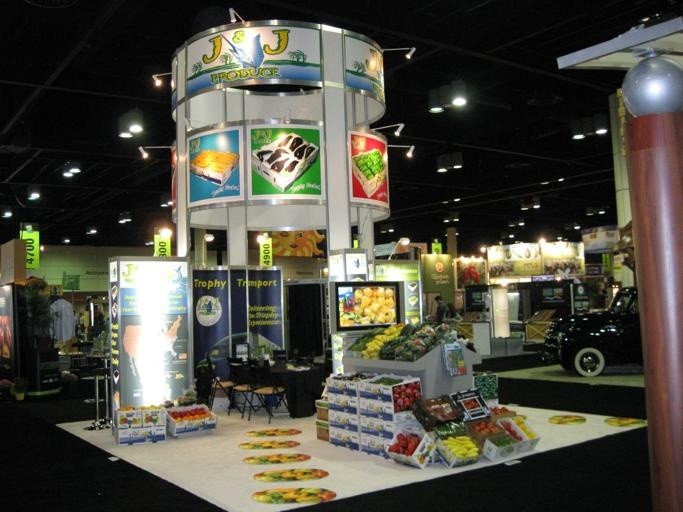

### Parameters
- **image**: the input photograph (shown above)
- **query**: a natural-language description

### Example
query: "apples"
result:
[389,434,421,456]
[503,420,523,440]
[170,407,206,418]
[393,382,421,412]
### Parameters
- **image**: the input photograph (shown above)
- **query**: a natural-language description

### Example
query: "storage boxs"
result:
[110,401,217,447]
[315,370,542,472]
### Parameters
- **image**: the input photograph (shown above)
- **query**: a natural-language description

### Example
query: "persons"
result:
[428,296,464,325]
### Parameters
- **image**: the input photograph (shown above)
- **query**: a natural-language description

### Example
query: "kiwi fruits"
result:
[474,421,499,434]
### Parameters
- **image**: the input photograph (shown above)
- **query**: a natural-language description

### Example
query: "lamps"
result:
[370,47,417,159]
[426,81,469,172]
[512,125,615,217]
[2,159,82,219]
[228,7,246,25]
[83,191,176,235]
[137,72,177,162]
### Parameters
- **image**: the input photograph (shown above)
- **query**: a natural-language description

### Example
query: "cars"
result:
[539,286,643,377]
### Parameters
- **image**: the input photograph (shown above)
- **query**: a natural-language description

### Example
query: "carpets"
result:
[489,355,646,388]
[52,394,648,512]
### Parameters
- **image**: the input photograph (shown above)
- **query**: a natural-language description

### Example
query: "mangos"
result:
[354,287,396,324]
[362,323,405,359]
[513,417,536,438]
[442,435,479,458]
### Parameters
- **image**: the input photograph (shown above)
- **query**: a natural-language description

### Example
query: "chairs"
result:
[206,351,292,424]
